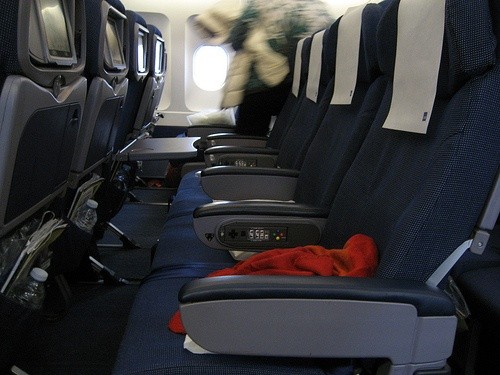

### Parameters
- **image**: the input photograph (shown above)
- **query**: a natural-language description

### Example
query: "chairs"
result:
[1,1,500,375]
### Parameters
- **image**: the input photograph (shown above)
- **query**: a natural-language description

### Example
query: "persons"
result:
[196,0,333,138]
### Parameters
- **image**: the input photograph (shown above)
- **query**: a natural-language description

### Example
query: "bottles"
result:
[72,200,98,232]
[113,176,127,191]
[9,268,48,310]
